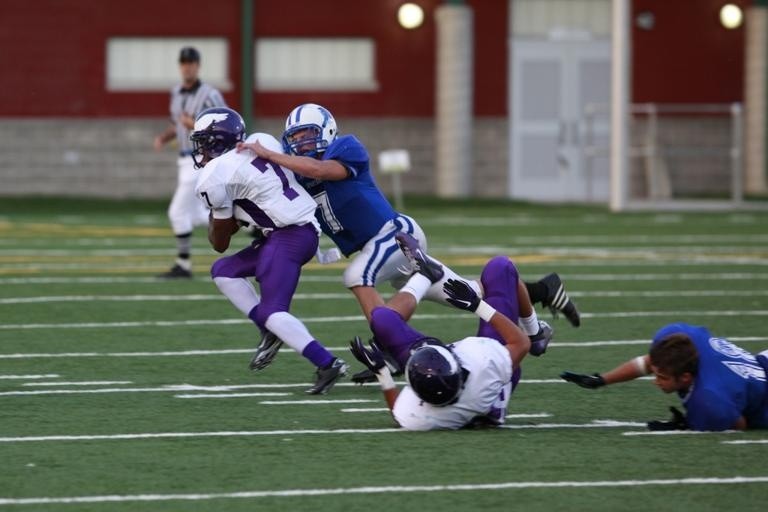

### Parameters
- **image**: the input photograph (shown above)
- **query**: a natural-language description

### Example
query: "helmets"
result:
[280,102,339,158]
[404,345,465,408]
[188,105,248,172]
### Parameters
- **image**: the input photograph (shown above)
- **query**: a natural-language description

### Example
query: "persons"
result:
[152,46,231,278]
[352,260,553,429]
[192,107,348,396]
[237,103,581,382]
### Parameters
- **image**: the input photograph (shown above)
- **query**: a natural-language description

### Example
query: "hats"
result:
[178,46,201,64]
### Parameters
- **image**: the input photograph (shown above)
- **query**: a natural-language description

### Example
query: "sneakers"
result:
[351,361,397,386]
[393,229,445,285]
[303,355,351,397]
[156,264,193,280]
[528,319,554,357]
[538,270,582,328]
[247,329,284,374]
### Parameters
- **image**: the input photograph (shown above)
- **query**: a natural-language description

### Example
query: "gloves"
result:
[646,404,691,432]
[348,332,396,392]
[558,369,606,390]
[440,277,497,324]
